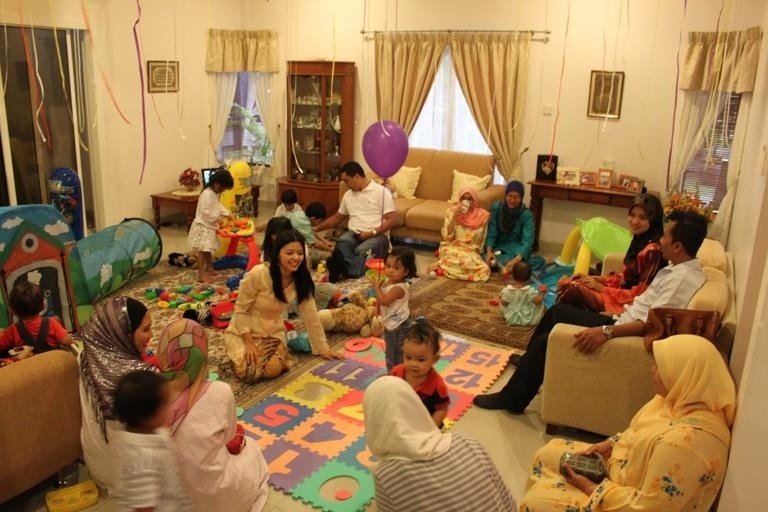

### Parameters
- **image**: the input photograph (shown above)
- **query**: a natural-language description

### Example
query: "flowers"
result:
[178,167,201,187]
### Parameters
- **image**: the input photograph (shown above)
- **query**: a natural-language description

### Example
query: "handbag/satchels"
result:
[644,307,722,354]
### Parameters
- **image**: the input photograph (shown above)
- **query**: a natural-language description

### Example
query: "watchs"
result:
[602,326,613,339]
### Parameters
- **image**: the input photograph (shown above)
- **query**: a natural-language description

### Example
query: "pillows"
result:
[388,165,423,199]
[447,169,492,204]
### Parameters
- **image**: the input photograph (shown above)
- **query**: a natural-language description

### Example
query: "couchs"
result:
[539,236,740,438]
[337,145,508,248]
[1,347,84,504]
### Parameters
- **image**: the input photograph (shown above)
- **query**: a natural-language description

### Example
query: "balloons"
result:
[362,120,408,179]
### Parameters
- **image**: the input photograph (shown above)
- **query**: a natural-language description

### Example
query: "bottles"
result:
[315,137,320,150]
[296,115,321,129]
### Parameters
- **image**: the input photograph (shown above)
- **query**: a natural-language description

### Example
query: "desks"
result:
[150,183,262,233]
[526,179,661,259]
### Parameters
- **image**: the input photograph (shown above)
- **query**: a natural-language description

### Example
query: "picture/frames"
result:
[585,69,627,119]
[554,166,646,196]
[146,59,181,94]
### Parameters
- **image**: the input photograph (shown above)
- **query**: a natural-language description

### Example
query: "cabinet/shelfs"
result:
[276,59,356,214]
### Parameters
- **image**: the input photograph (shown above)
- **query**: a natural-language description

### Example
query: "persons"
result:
[518,334,735,512]
[473,205,707,413]
[558,193,664,315]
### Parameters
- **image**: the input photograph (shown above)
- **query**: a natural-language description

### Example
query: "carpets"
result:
[90,248,436,413]
[404,265,554,351]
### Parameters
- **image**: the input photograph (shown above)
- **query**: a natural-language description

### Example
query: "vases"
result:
[185,183,195,191]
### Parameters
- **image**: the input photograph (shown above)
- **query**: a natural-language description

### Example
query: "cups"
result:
[296,94,341,105]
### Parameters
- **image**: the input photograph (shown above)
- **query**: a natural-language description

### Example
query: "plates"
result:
[303,136,314,151]
